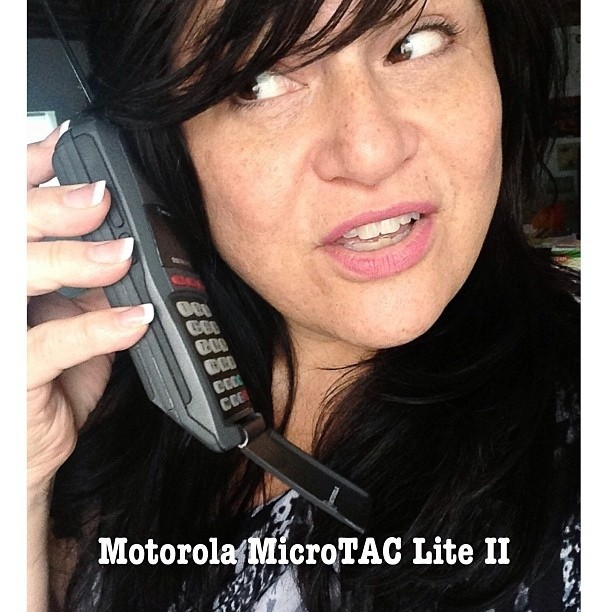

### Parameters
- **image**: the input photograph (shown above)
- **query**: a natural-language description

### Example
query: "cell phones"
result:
[39,1,376,536]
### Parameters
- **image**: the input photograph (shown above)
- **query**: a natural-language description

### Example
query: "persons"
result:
[26,0,581,611]
